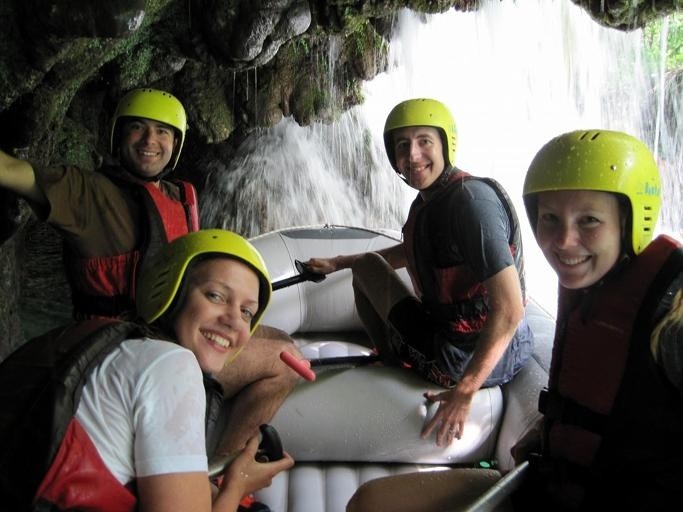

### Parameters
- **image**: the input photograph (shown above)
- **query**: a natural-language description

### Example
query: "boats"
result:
[205,224,551,512]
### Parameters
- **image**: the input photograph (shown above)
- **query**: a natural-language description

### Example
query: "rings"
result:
[447,427,456,432]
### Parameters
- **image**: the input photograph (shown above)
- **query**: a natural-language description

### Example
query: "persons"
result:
[345,129,681,511]
[0,228,295,512]
[0,87,307,512]
[304,98,534,445]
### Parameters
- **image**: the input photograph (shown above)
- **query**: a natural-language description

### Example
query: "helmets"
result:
[110,87,188,173]
[520,127,663,259]
[382,97,459,171]
[133,226,274,368]
